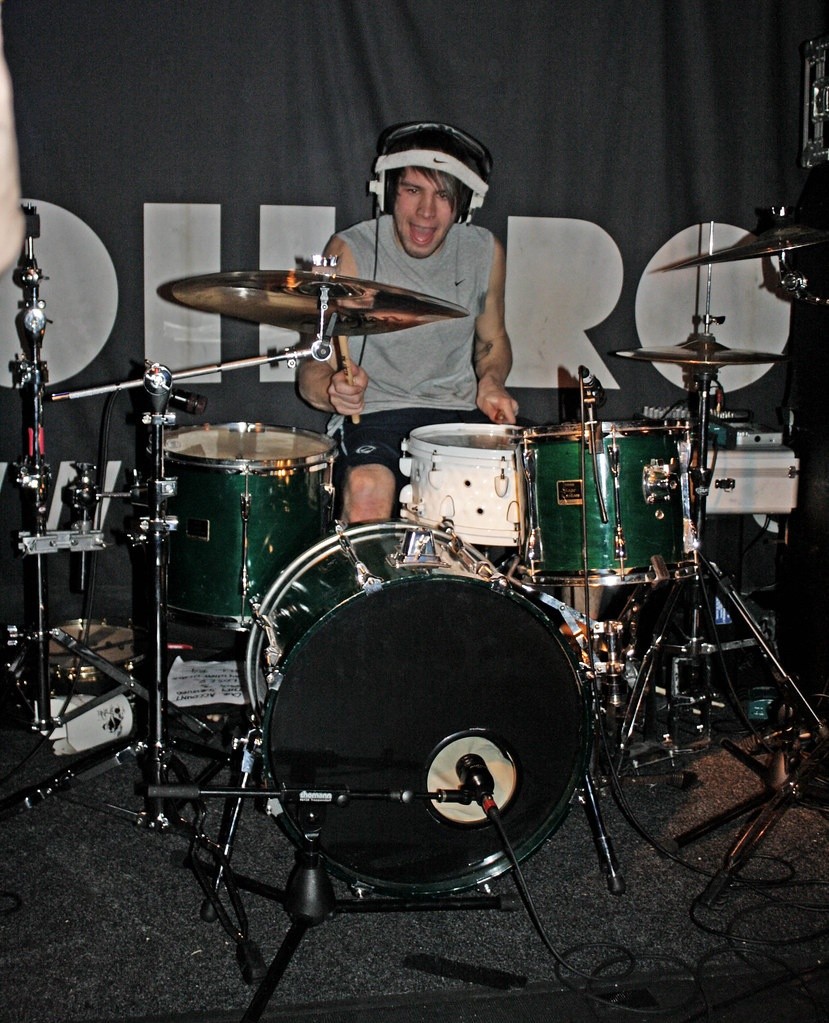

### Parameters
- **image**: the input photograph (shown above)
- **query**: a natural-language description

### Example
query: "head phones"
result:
[364,122,494,228]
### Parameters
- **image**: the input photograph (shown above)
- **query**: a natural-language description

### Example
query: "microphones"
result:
[165,386,208,417]
[455,753,495,802]
[578,364,608,410]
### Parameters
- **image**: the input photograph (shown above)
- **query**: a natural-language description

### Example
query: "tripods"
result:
[0,211,829,1022]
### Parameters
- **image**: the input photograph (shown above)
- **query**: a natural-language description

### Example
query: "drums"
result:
[517,415,701,590]
[244,521,594,897]
[399,421,528,549]
[147,418,340,634]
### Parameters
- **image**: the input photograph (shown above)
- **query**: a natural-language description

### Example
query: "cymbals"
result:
[650,202,829,277]
[156,251,473,336]
[612,332,799,367]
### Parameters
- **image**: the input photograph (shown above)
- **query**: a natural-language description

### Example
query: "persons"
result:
[0,22,25,280]
[298,121,605,622]
